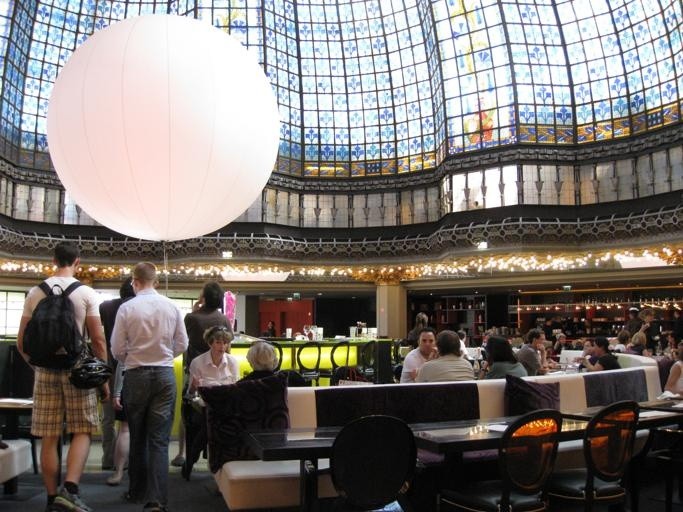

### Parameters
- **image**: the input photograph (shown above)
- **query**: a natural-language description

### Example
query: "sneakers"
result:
[123,490,148,505]
[45,487,94,512]
[142,500,168,512]
[171,456,186,467]
[106,472,124,485]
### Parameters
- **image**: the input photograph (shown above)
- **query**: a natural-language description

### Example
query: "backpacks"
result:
[22,279,87,371]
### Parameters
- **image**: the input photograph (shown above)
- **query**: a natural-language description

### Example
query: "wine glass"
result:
[303,325,317,341]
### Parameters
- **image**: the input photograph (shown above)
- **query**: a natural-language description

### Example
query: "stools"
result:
[1,437,36,498]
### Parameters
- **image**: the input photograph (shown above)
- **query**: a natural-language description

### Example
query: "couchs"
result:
[559,350,658,368]
[386,376,570,484]
[533,366,663,415]
[198,384,392,512]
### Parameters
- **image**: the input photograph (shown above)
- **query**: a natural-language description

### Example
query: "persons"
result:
[15,240,109,511]
[110,261,188,512]
[170,282,233,466]
[188,326,240,400]
[98,277,136,469]
[104,359,132,485]
[234,341,278,389]
[261,321,276,338]
[398,307,683,399]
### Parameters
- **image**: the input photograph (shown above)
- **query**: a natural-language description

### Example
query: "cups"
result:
[286,328,292,338]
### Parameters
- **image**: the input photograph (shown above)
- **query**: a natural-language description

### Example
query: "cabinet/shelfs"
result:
[411,293,489,338]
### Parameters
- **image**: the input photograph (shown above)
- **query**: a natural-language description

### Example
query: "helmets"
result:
[69,359,115,390]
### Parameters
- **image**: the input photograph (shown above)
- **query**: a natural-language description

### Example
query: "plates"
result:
[259,336,295,342]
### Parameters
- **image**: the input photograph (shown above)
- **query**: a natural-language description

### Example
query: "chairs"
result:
[6,343,42,474]
[633,416,683,512]
[436,400,640,512]
[316,415,417,512]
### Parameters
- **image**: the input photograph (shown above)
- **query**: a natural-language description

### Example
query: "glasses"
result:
[208,326,229,336]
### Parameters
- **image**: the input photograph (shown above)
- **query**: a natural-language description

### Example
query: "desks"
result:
[545,362,591,377]
[402,412,591,492]
[561,399,683,472]
[300,366,375,386]
[0,398,39,441]
[246,426,343,512]
